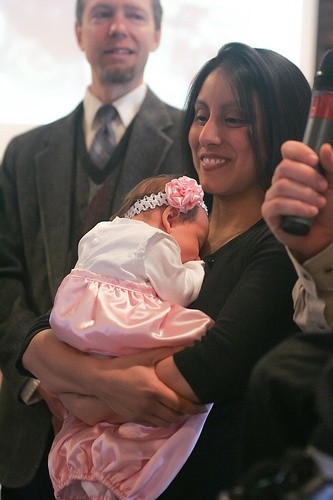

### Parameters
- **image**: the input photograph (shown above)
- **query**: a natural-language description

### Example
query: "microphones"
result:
[279,49,333,236]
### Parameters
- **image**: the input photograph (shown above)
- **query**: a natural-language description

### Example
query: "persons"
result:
[235,141,333,500]
[46,173,216,500]
[0,0,186,500]
[18,41,314,500]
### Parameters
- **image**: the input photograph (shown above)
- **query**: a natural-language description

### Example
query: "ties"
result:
[90,105,121,171]
